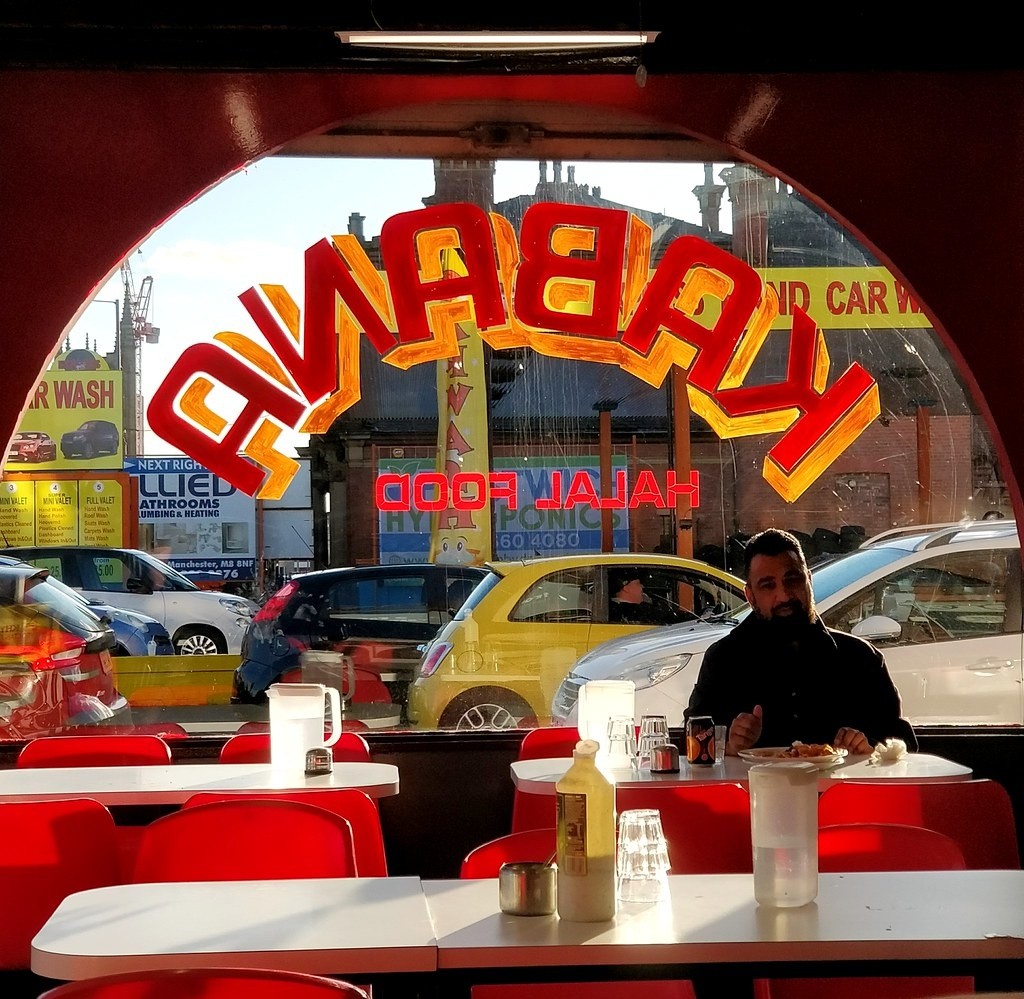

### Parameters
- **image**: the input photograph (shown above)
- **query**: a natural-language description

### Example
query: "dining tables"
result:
[28,868,1023,998]
[510,752,975,796]
[0,761,401,805]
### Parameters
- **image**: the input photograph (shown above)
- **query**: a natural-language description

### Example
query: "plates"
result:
[737,746,850,760]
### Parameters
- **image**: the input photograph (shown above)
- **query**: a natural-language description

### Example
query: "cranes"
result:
[118,258,160,460]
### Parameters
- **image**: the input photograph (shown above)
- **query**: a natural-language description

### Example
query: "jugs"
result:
[265,681,341,763]
[574,679,637,770]
[751,759,822,909]
[298,648,356,714]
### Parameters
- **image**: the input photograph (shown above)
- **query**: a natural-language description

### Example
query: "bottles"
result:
[554,742,616,920]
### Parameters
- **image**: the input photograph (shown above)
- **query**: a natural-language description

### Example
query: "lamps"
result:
[336,30,662,51]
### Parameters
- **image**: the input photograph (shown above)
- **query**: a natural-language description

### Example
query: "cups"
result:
[499,860,557,916]
[651,743,679,773]
[305,748,332,773]
[617,811,672,902]
[714,725,727,763]
[636,715,669,770]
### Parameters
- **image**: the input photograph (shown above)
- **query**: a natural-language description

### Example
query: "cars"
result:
[237,558,490,704]
[8,431,56,463]
[60,420,119,458]
[46,574,174,658]
[0,553,134,738]
[406,551,749,731]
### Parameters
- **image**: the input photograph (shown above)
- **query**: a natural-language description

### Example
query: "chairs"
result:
[614,784,753,877]
[36,967,373,999]
[16,735,172,770]
[511,726,640,837]
[132,799,374,997]
[818,778,1018,868]
[0,798,118,971]
[183,790,389,881]
[754,823,975,999]
[460,829,697,999]
[219,733,372,764]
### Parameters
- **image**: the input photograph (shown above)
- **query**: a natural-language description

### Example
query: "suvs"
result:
[549,517,1024,730]
[1,542,262,656]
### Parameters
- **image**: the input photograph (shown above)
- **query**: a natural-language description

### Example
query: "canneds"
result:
[687,716,716,769]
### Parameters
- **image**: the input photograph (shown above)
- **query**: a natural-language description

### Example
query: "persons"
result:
[681,526,918,757]
[609,568,683,627]
[448,578,473,612]
